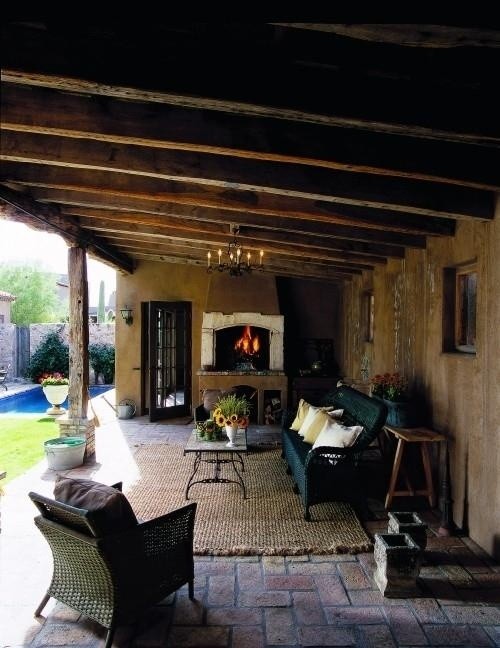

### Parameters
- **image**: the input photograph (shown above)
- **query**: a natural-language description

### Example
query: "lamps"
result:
[206,226,266,278]
[120,304,133,325]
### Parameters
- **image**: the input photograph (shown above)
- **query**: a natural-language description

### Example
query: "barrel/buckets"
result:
[43,437,86,470]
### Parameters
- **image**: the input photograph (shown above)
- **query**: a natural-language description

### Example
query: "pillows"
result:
[289,398,364,465]
[53,478,138,532]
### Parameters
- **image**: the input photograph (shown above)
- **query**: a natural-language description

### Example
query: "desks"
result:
[382,426,448,510]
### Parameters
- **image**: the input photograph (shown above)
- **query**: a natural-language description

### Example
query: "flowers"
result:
[211,393,252,429]
[38,370,69,388]
[368,369,409,401]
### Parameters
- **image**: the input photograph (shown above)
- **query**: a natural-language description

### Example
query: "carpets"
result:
[101,444,376,557]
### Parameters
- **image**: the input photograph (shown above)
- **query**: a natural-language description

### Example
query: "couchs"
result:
[281,383,387,523]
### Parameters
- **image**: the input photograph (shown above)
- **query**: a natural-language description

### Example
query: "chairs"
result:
[26,479,201,647]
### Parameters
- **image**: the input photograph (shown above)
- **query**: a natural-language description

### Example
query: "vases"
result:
[368,390,415,428]
[44,384,68,415]
[224,424,240,448]
